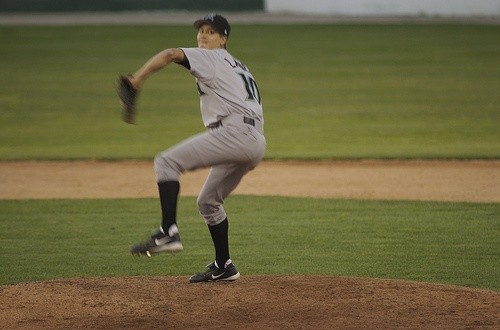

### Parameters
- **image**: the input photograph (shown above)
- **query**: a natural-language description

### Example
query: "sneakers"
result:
[130,223,183,257]
[188,258,239,284]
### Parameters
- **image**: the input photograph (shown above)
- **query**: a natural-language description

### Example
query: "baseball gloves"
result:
[114,74,141,124]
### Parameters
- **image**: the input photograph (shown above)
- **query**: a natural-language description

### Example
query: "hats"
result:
[193,13,231,38]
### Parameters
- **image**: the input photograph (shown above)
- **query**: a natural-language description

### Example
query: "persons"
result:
[116,13,267,282]
